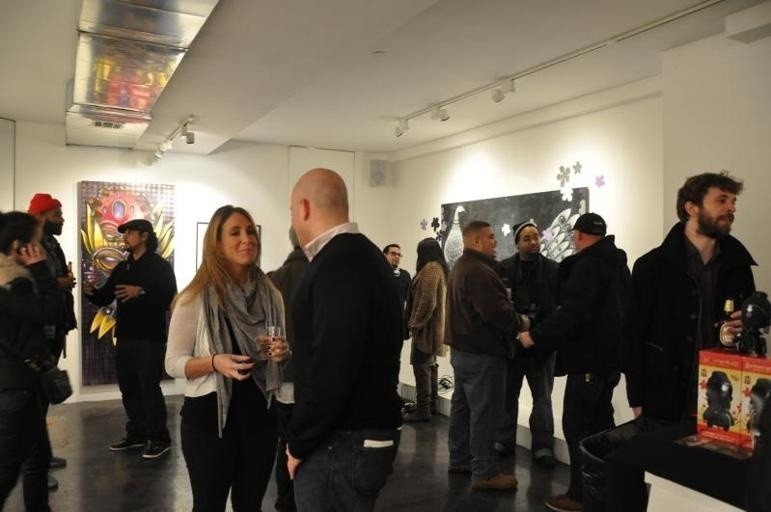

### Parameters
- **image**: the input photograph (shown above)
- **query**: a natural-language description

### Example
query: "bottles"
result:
[64,261,74,292]
[717,298,739,351]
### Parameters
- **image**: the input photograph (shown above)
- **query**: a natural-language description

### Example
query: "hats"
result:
[512,222,537,245]
[27,193,62,215]
[117,219,153,234]
[569,213,606,236]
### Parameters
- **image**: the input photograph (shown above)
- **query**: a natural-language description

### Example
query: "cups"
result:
[258,327,282,360]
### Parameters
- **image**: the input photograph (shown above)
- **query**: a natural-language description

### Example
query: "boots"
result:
[403,367,432,421]
[432,365,439,415]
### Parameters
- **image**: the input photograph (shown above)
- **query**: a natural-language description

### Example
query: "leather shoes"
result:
[530,445,557,468]
[494,438,517,456]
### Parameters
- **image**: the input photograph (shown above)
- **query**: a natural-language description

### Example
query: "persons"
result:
[619,169,759,417]
[704,369,771,433]
[516,212,632,511]
[284,167,405,512]
[162,202,291,512]
[497,220,561,468]
[264,224,312,512]
[0,209,54,512]
[443,220,531,490]
[382,244,412,411]
[407,237,450,422]
[20,193,78,493]
[82,218,178,461]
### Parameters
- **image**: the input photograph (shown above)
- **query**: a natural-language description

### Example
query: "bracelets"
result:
[139,287,146,297]
[211,353,219,373]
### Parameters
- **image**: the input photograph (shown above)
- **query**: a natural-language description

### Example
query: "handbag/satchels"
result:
[38,369,73,404]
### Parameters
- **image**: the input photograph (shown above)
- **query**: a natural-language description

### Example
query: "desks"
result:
[636,435,770,512]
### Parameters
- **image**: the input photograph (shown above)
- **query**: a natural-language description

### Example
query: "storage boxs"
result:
[695,338,771,450]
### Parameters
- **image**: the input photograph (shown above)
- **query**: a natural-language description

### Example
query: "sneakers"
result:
[46,472,61,491]
[545,494,586,512]
[50,453,65,469]
[448,460,470,469]
[142,439,170,459]
[471,474,518,491]
[109,434,146,451]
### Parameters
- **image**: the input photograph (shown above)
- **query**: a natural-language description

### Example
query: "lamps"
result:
[393,70,514,144]
[151,114,194,159]
